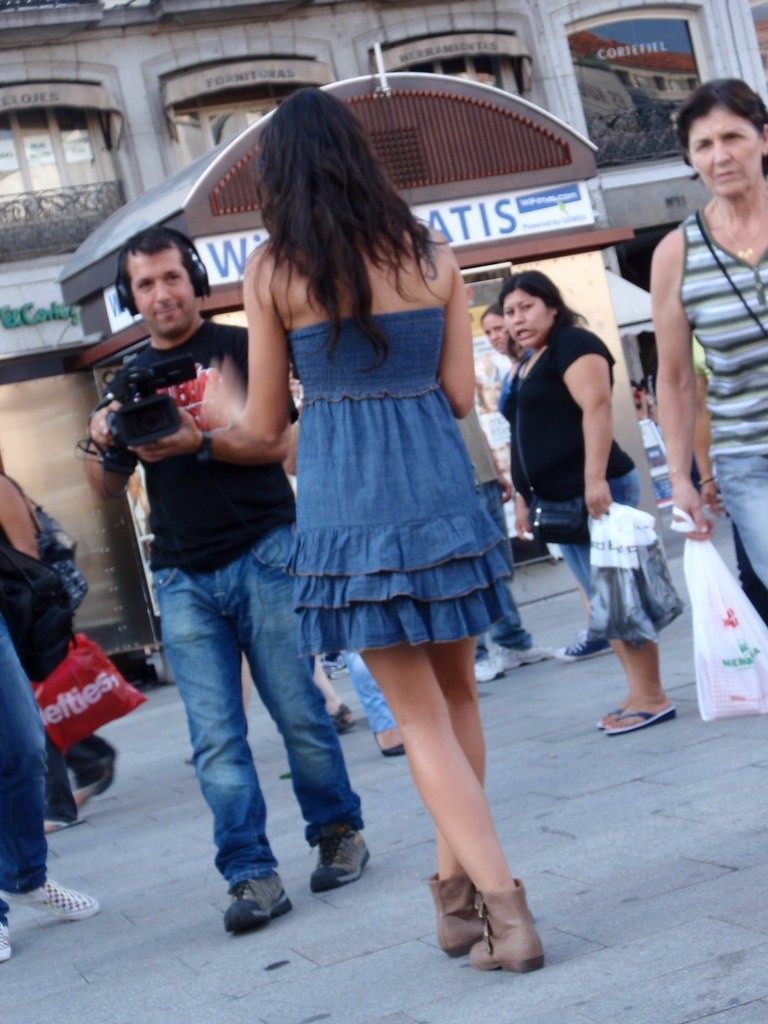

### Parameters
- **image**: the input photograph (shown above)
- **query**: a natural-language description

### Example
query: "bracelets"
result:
[697,476,716,490]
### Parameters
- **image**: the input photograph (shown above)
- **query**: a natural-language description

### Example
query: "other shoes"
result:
[71,768,111,809]
[317,649,350,680]
[375,727,405,756]
[0,923,11,961]
[0,879,99,920]
[44,816,85,834]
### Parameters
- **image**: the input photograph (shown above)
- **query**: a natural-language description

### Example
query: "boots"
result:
[468,879,545,974]
[429,872,488,958]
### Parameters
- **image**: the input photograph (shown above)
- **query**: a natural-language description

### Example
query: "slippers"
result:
[596,709,625,731]
[604,706,677,737]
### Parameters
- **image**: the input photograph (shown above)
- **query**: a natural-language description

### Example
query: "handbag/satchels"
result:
[584,501,684,645]
[28,632,148,755]
[533,497,585,544]
[671,506,768,721]
[33,506,89,613]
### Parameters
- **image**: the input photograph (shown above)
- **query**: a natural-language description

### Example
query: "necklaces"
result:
[713,195,766,260]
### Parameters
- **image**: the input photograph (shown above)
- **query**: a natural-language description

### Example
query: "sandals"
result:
[329,703,356,733]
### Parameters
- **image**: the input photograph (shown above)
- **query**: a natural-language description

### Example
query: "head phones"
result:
[114,227,207,316]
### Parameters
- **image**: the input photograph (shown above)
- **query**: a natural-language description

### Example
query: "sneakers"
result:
[224,875,293,931]
[310,818,370,893]
[555,628,612,663]
[472,660,505,682]
[495,643,555,670]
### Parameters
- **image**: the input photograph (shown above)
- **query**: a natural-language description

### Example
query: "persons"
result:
[280,302,615,756]
[85,224,372,933]
[502,270,678,735]
[0,452,116,959]
[631,332,768,630]
[649,80,768,588]
[197,89,548,975]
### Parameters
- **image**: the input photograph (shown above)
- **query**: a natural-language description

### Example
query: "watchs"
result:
[195,432,213,461]
